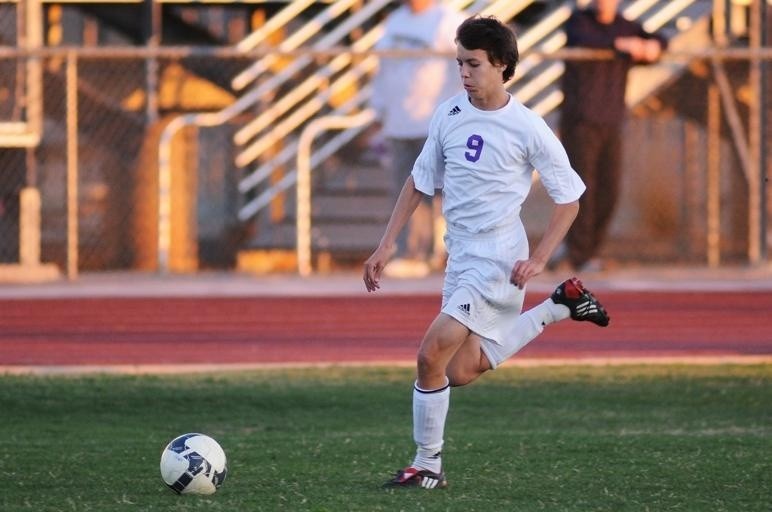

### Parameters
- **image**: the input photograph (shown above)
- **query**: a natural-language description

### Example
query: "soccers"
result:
[160,432,227,495]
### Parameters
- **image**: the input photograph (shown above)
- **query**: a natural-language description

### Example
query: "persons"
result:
[556,0,667,276]
[372,0,471,280]
[363,17,610,490]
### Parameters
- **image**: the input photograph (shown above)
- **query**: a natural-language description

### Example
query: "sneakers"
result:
[379,460,448,490]
[551,276,610,329]
